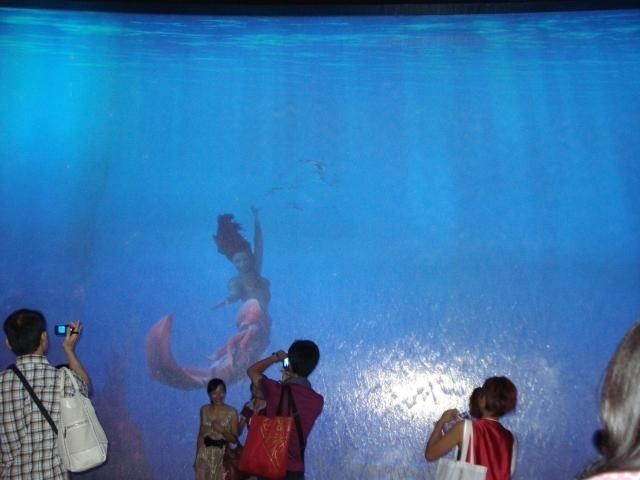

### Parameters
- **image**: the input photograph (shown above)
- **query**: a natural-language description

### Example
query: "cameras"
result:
[54,324,71,336]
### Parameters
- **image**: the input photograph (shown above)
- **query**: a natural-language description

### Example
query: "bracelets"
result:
[272,352,279,361]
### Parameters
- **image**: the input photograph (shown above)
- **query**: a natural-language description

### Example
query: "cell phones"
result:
[283,353,289,369]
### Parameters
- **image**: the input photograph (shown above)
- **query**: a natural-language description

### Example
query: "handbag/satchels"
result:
[434,458,489,480]
[223,413,294,480]
[56,390,112,474]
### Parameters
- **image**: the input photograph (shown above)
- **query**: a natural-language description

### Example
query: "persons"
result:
[1,308,93,480]
[232,382,268,435]
[243,339,325,480]
[191,377,241,480]
[142,203,272,391]
[424,376,519,479]
[570,320,640,479]
